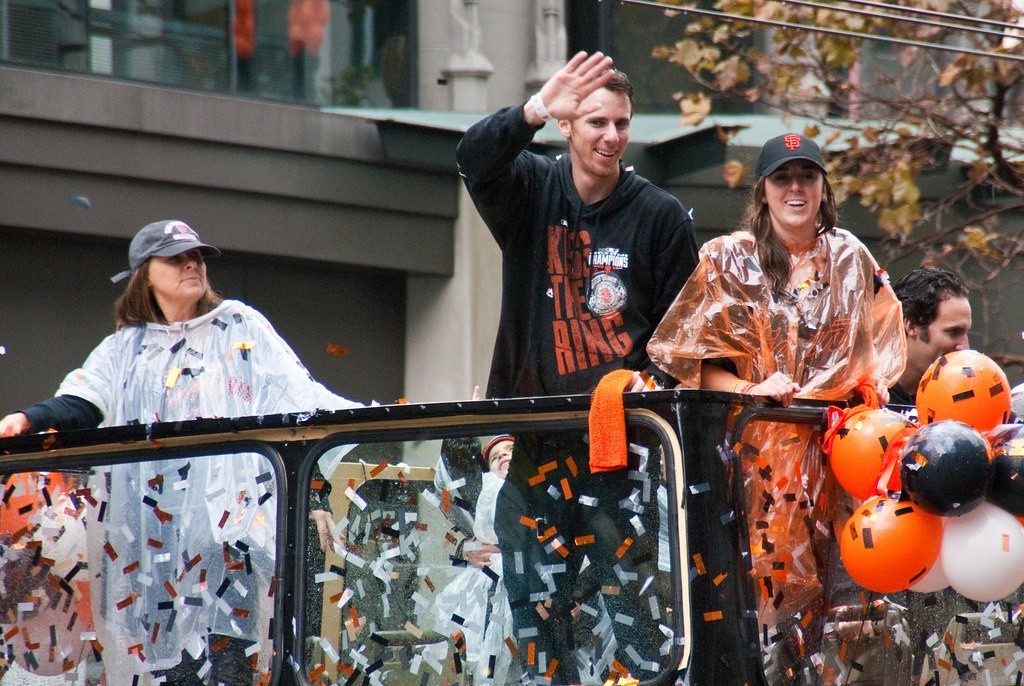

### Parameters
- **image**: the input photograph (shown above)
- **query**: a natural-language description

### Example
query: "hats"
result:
[128,218,222,274]
[753,132,828,178]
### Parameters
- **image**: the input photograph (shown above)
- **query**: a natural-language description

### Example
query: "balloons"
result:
[840,480,942,594]
[899,503,959,594]
[913,345,1013,457]
[901,416,990,519]
[830,409,934,504]
[978,422,1024,520]
[942,486,1024,603]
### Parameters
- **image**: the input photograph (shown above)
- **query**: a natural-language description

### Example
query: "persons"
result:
[1,216,382,686]
[819,258,976,679]
[447,45,703,684]
[644,130,909,684]
[432,385,523,686]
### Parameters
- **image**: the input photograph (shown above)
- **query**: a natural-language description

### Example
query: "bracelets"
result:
[529,90,559,127]
